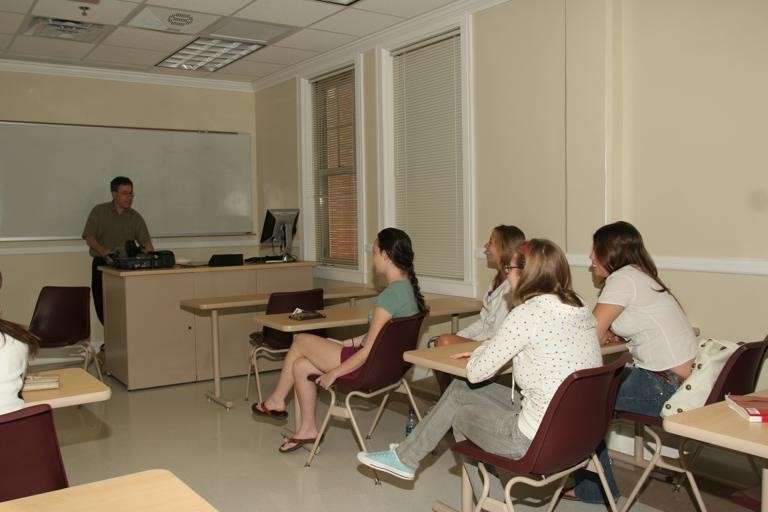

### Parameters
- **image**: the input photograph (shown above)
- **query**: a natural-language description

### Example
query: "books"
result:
[725,395,768,423]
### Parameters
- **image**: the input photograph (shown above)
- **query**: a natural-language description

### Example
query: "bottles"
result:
[405,408,416,438]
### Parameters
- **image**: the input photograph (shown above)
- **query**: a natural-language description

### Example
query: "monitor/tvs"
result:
[260,209,299,264]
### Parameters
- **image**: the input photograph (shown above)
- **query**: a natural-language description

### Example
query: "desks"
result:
[100,261,323,389]
[663,390,768,459]
[255,293,483,452]
[21,367,112,408]
[180,287,382,408]
[0,469,218,512]
[403,340,701,512]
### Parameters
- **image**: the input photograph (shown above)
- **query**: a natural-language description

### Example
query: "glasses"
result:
[503,265,521,278]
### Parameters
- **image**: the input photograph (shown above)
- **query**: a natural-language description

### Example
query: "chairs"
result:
[1,405,69,502]
[246,289,324,401]
[432,354,630,512]
[306,308,437,486]
[610,340,768,512]
[27,285,104,409]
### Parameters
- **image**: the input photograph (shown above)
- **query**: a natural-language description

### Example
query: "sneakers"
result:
[356,443,420,481]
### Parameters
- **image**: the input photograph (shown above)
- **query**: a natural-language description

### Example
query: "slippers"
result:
[279,432,316,453]
[560,485,618,504]
[252,400,289,419]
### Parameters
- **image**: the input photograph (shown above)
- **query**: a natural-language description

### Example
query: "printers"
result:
[112,240,175,270]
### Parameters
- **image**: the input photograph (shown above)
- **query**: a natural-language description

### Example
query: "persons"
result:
[0,273,42,415]
[256,227,429,451]
[82,176,154,327]
[560,222,698,504]
[357,238,602,503]
[434,225,526,396]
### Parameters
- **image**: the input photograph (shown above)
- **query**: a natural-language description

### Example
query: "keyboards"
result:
[245,256,284,264]
